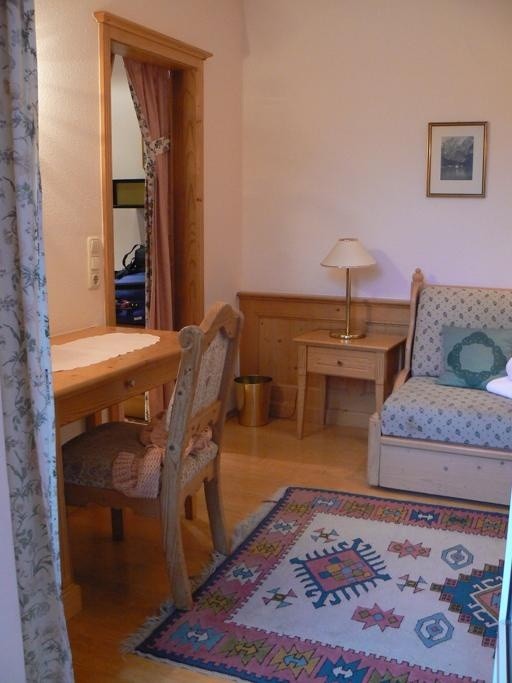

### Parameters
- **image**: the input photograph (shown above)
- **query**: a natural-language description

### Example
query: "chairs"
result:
[56,300,256,614]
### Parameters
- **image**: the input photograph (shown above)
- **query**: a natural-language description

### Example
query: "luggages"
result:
[116,273,145,324]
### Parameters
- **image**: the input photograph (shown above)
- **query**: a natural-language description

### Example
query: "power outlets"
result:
[86,271,104,287]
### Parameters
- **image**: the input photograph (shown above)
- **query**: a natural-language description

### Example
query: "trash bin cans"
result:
[233,375,272,427]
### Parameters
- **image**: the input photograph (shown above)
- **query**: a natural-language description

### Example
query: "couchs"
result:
[363,266,511,508]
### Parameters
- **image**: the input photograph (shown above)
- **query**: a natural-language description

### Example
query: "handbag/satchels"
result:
[135,245,145,273]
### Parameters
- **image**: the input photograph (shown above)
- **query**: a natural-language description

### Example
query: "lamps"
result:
[319,237,378,340]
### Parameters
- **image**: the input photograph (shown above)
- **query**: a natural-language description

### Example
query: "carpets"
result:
[118,482,512,683]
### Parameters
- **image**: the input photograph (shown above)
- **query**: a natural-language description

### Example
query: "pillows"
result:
[434,320,511,393]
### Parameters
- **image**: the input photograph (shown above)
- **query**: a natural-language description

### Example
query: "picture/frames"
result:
[425,120,489,198]
[110,178,146,209]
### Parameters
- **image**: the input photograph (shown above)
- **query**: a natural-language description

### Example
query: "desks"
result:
[44,319,205,622]
[290,326,407,446]
